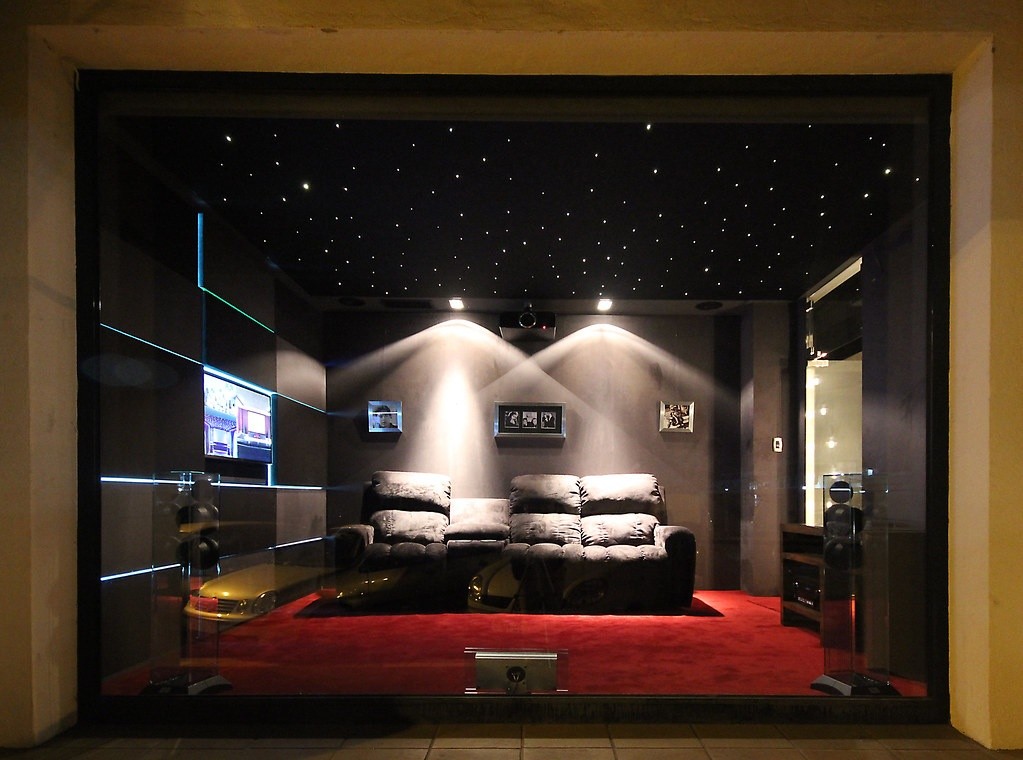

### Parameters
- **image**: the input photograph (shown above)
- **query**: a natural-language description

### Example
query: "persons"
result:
[371,406,398,428]
[505,411,555,427]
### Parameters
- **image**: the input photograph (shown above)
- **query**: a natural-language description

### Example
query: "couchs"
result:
[330,470,451,607]
[510,473,697,614]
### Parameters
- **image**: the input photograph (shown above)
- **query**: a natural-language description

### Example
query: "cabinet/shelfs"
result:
[779,519,864,648]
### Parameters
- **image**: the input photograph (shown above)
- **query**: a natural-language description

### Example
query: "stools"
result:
[445,497,511,609]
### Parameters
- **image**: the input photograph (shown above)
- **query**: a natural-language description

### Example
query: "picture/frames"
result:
[660,401,695,433]
[368,400,403,432]
[494,401,568,438]
